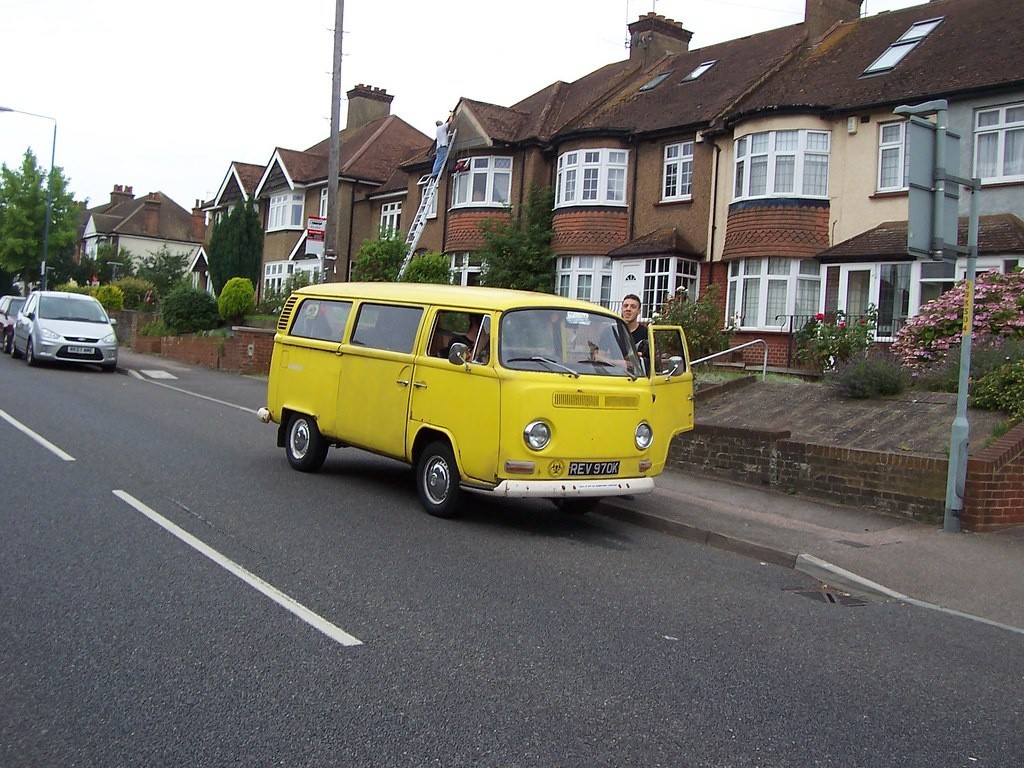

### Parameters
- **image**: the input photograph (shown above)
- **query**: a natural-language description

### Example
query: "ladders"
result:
[396,129,459,279]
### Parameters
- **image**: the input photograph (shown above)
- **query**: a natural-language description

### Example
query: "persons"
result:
[431,111,454,180]
[594,294,650,370]
[447,312,489,362]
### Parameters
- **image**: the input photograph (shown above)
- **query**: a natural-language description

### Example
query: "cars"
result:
[10,291,120,372]
[0,295,26,354]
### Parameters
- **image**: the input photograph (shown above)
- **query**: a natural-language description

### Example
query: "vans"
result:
[253,280,697,519]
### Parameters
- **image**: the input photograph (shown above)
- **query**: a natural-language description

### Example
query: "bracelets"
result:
[450,116,453,117]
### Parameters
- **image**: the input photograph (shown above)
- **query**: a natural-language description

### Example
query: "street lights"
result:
[0,105,59,291]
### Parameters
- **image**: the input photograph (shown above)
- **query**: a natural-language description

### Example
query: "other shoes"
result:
[617,494,635,501]
